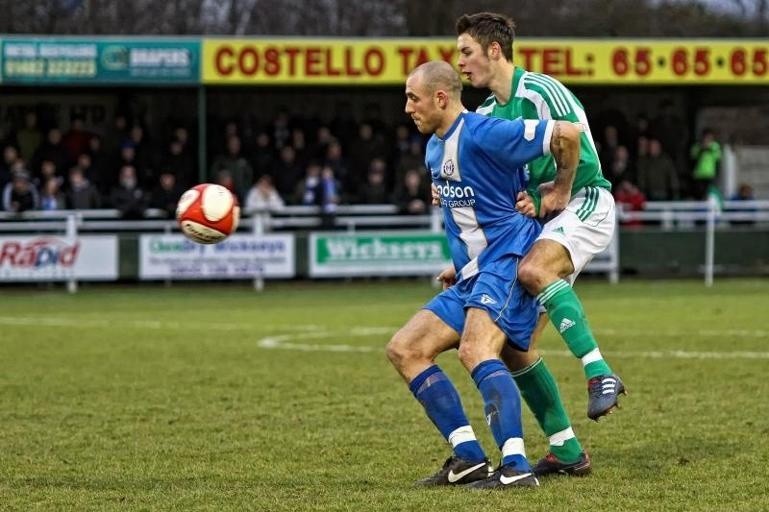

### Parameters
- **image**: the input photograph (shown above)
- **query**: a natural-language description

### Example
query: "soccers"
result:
[176,183,241,244]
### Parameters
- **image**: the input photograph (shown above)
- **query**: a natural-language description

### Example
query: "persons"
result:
[584,104,758,234]
[1,103,445,237]
[435,12,629,480]
[383,58,584,494]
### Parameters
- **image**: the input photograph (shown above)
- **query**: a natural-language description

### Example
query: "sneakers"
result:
[534,449,592,476]
[420,455,493,488]
[586,372,627,423]
[471,463,540,491]
